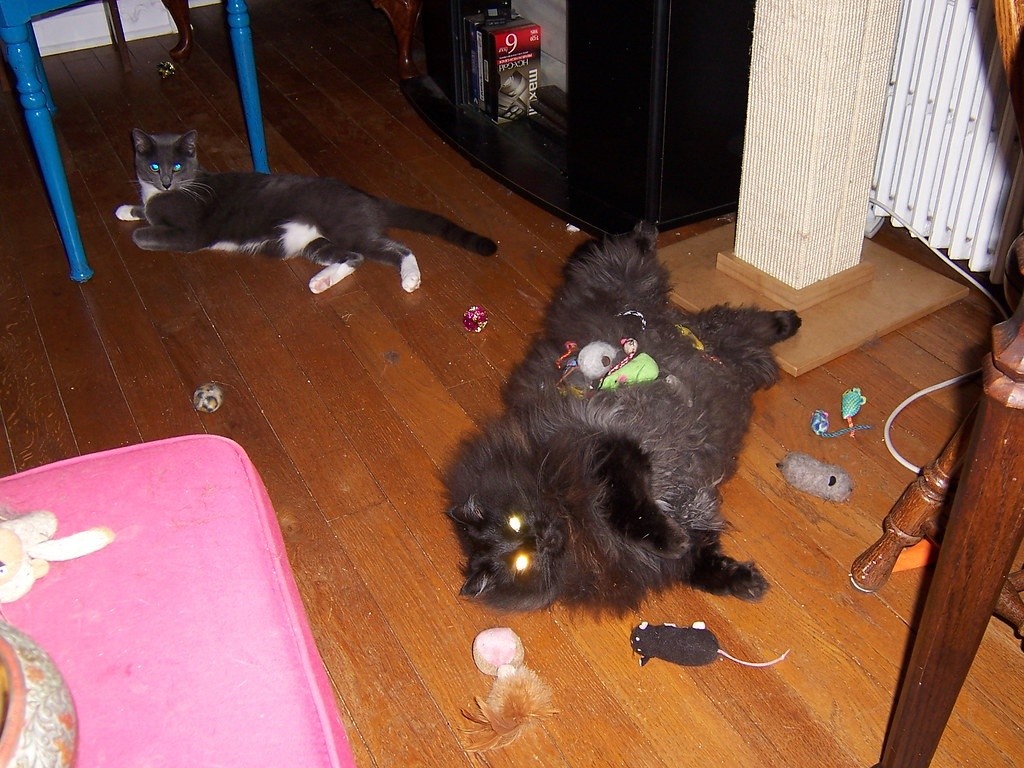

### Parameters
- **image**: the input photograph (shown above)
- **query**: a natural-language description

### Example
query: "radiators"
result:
[863,1,1024,284]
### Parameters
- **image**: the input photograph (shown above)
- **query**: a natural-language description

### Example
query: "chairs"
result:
[848,0,1024,767]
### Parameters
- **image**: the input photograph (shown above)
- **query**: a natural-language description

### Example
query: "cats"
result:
[441,223,805,618]
[113,128,500,296]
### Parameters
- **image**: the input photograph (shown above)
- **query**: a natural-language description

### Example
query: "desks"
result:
[0,0,272,284]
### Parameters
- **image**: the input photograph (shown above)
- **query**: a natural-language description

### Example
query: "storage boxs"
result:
[462,11,541,126]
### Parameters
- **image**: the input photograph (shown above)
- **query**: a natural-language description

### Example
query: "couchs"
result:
[0,428,354,768]
